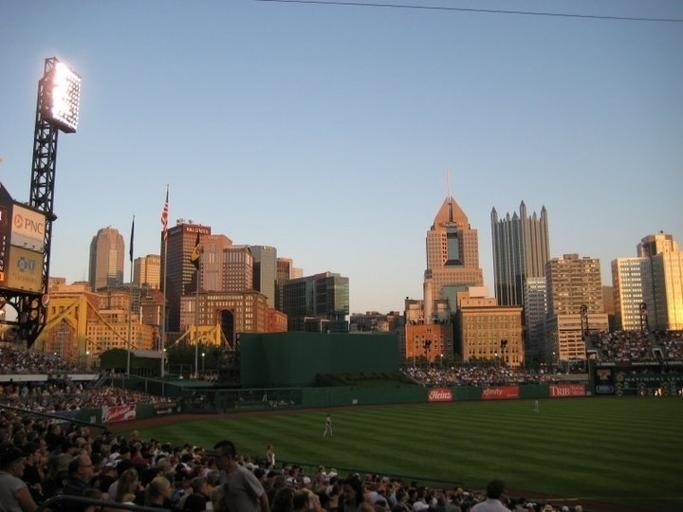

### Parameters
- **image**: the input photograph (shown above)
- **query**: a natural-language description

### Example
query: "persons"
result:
[1,329,682,512]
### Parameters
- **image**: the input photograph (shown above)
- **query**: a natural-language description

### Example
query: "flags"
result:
[190,231,199,270]
[160,186,169,240]
[129,218,134,261]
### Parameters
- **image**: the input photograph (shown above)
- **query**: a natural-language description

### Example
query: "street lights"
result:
[422,338,430,382]
[498,338,509,383]
